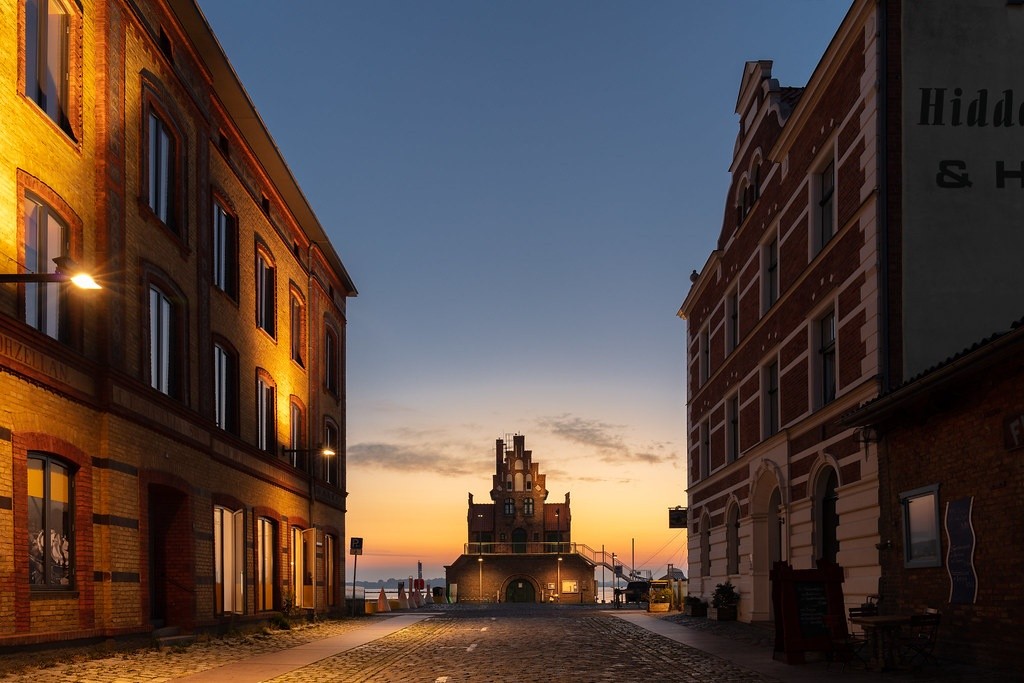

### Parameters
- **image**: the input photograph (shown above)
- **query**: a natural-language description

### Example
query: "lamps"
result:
[0,256,104,291]
[282,444,336,457]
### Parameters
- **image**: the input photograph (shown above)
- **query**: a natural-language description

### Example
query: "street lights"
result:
[555,507,563,603]
[477,513,485,604]
[612,553,617,607]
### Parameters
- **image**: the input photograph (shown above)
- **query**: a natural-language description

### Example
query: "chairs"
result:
[897,615,940,666]
[821,614,868,673]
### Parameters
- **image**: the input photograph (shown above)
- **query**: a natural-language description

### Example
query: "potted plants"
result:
[680,596,708,618]
[648,588,673,614]
[710,581,741,621]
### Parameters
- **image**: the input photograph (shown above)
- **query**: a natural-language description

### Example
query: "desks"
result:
[848,616,911,671]
